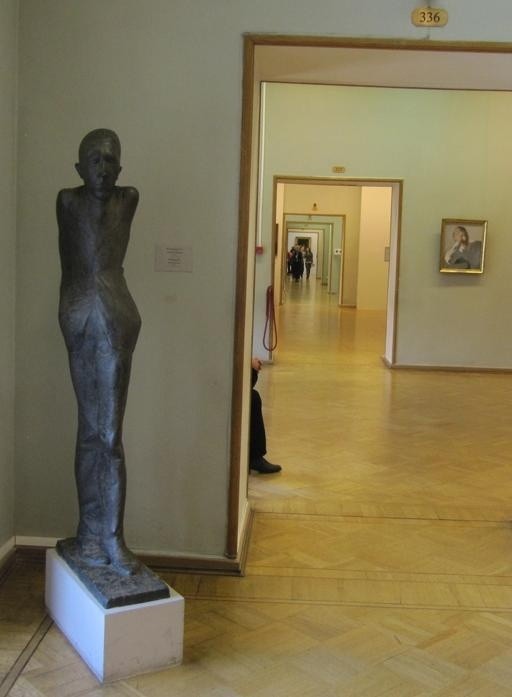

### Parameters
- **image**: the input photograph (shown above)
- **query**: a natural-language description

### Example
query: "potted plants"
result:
[439,218,488,274]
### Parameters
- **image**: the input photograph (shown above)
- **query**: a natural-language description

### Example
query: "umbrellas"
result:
[250,458,281,473]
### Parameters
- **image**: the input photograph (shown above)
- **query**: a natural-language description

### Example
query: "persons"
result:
[247,356,284,476]
[49,128,145,575]
[443,226,483,270]
[285,242,316,281]
